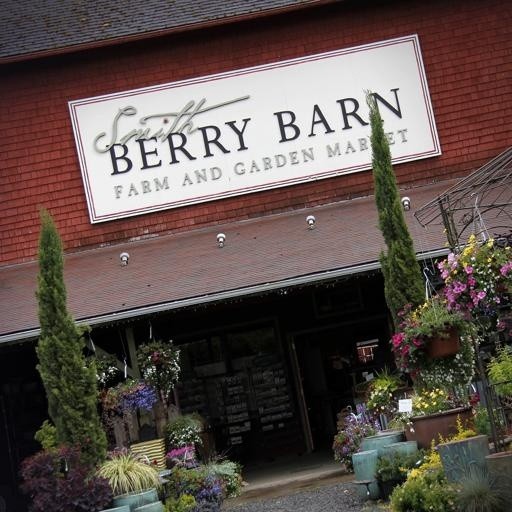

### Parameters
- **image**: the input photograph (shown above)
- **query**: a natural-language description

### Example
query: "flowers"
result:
[104,342,202,469]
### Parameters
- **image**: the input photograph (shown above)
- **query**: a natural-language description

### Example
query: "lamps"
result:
[401,196,411,211]
[120,252,130,267]
[305,215,316,230]
[217,233,226,248]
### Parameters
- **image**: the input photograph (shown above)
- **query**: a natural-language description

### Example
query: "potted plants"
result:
[95,446,162,509]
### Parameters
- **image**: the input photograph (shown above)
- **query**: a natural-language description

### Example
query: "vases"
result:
[353,409,489,501]
